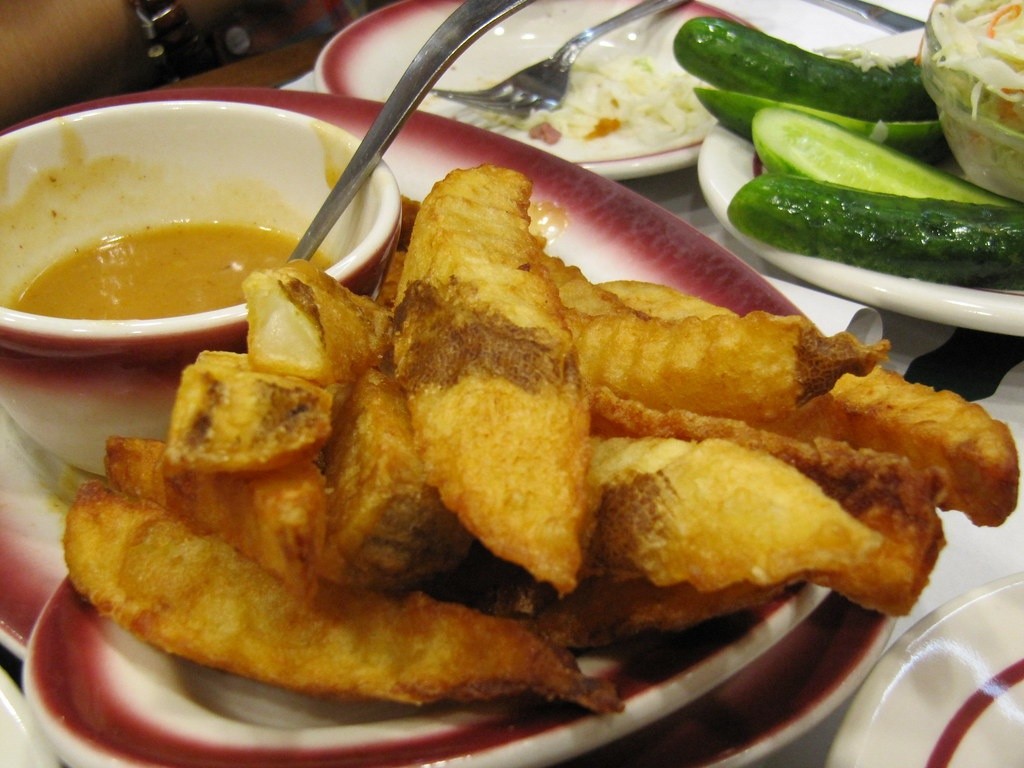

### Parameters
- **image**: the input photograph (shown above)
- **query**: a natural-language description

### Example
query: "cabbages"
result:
[927,0,1024,124]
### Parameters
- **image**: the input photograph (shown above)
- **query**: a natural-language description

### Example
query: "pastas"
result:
[481,53,723,148]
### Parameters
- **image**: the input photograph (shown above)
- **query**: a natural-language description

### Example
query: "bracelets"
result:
[134,0,206,65]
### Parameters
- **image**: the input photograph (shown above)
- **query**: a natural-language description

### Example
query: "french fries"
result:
[58,165,1021,714]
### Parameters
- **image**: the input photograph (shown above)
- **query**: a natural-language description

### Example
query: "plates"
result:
[313,0,766,180]
[18,567,835,768]
[0,125,897,768]
[824,570,1024,768]
[695,24,1024,337]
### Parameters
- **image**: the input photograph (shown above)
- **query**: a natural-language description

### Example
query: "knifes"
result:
[799,0,927,35]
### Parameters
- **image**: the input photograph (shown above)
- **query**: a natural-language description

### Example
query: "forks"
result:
[426,0,709,118]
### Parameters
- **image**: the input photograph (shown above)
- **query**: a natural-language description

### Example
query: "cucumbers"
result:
[673,14,1024,287]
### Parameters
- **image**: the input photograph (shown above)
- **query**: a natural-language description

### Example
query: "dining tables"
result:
[0,33,1024,768]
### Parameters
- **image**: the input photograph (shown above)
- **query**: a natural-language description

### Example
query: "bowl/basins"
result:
[917,0,1024,203]
[0,97,404,370]
[924,0,1024,135]
